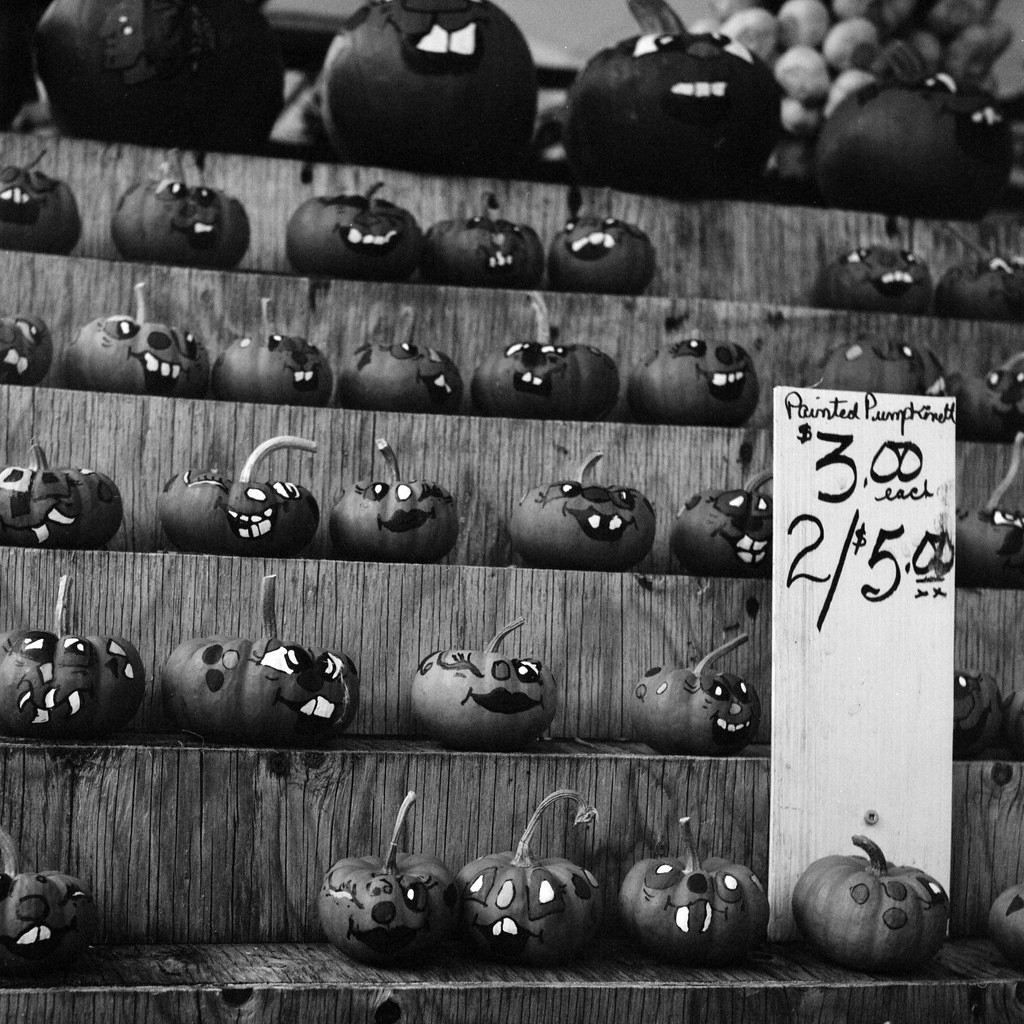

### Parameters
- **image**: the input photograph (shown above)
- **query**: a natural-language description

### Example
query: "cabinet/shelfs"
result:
[0,0,1024,1024]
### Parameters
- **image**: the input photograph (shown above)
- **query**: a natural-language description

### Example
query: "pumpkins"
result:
[0,0,1024,971]
